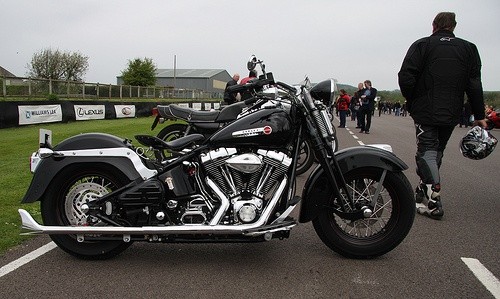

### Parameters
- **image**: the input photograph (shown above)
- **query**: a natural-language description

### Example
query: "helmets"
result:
[459,125,498,161]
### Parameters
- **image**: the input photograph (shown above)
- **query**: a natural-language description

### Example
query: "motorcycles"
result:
[151,72,314,176]
[20,56,416,260]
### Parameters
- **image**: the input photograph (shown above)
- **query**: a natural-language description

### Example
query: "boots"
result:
[415,183,445,219]
[413,180,428,203]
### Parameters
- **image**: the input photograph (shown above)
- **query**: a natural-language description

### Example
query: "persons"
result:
[331,80,500,134]
[398,12,488,220]
[224,70,261,104]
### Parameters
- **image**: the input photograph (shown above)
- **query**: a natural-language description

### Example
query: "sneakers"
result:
[365,130,370,134]
[359,129,365,133]
[338,124,345,128]
